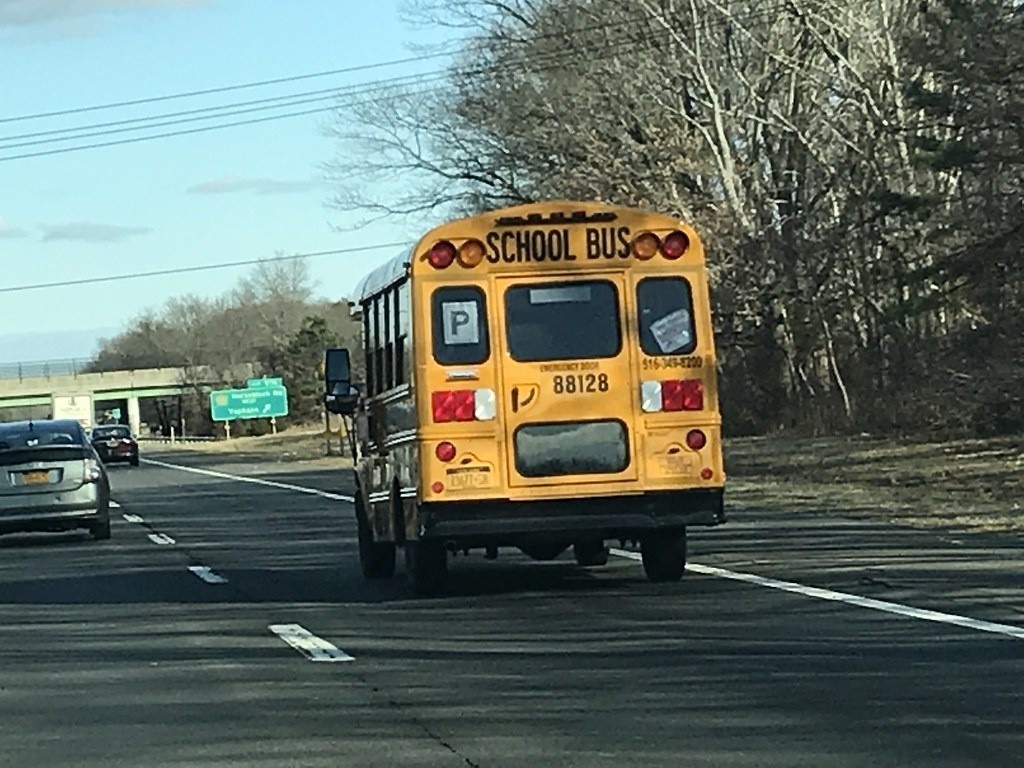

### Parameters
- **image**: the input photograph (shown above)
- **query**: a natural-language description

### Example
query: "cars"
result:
[87,424,141,466]
[0,419,113,541]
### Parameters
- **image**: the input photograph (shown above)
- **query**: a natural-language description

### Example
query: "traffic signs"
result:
[209,386,289,420]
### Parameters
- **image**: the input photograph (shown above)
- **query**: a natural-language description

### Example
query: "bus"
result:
[320,203,729,595]
